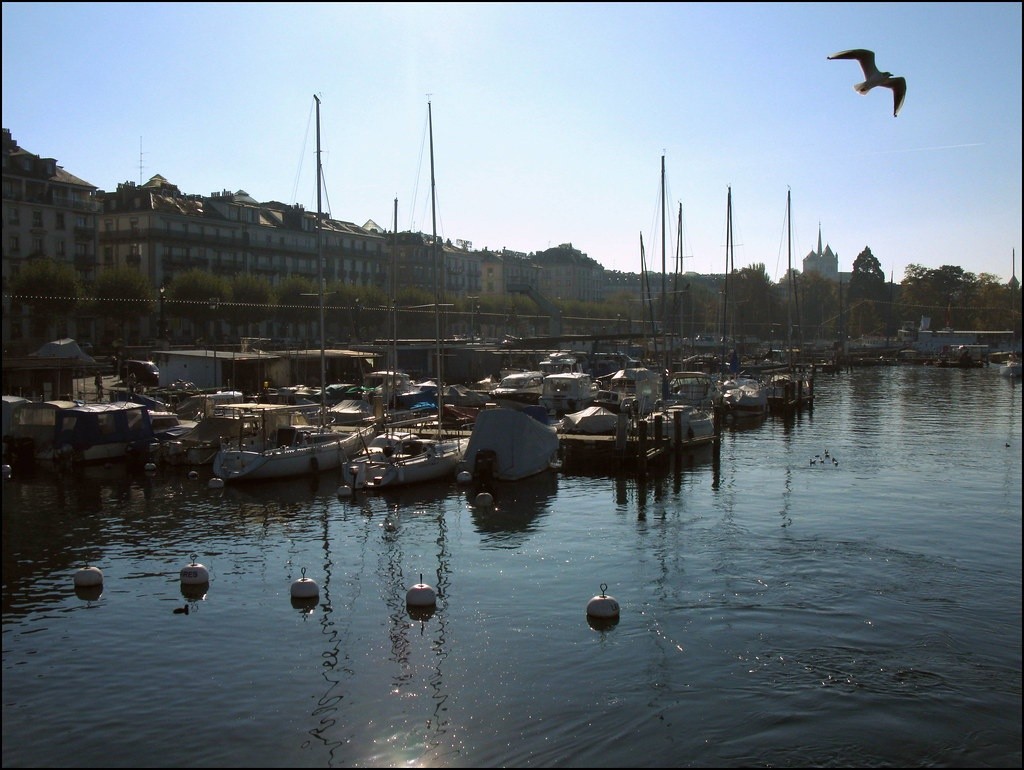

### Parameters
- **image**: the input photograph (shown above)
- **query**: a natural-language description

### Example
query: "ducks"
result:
[1004,441,1010,448]
[809,447,839,469]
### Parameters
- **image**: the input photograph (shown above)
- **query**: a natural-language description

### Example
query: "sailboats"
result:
[0,93,1023,497]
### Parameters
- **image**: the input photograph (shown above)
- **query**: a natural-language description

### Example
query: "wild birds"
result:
[827,48,907,118]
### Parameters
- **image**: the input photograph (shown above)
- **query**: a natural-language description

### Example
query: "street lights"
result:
[354,298,358,344]
[160,283,165,342]
[466,296,479,342]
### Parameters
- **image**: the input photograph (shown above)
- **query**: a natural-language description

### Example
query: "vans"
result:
[119,360,159,384]
